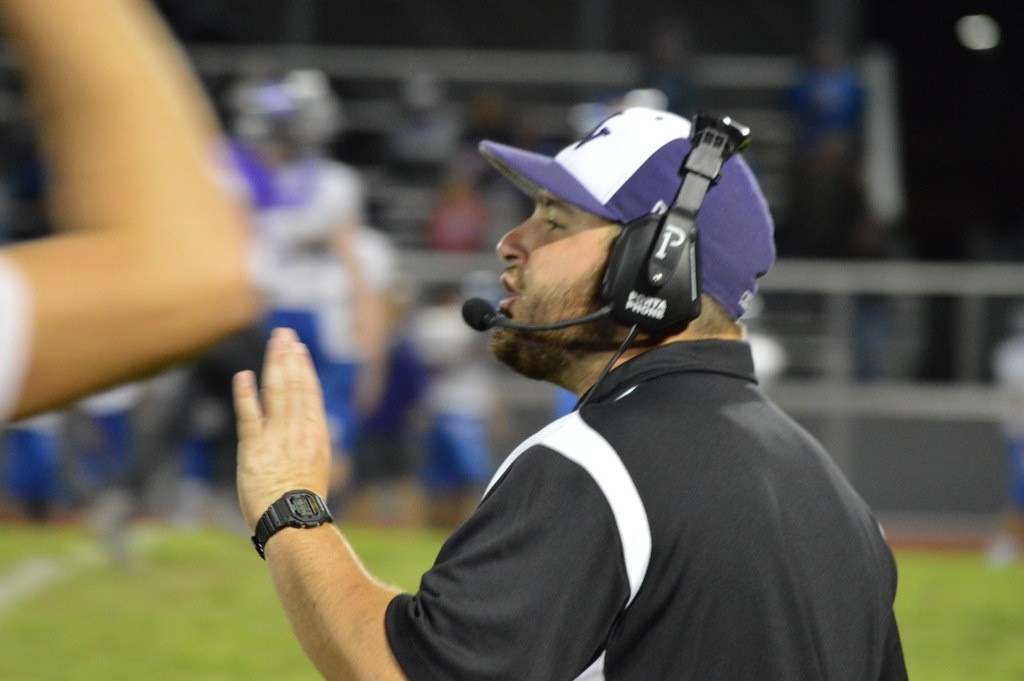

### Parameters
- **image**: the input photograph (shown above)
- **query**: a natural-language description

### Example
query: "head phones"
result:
[600,111,755,338]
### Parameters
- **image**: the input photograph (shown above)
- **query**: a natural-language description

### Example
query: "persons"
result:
[0,1,1024,567]
[232,106,909,681]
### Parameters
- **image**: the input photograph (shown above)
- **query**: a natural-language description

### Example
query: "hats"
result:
[478,102,776,319]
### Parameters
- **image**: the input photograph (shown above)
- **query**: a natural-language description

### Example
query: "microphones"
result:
[462,297,613,332]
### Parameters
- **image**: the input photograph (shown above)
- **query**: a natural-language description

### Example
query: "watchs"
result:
[251,489,334,560]
